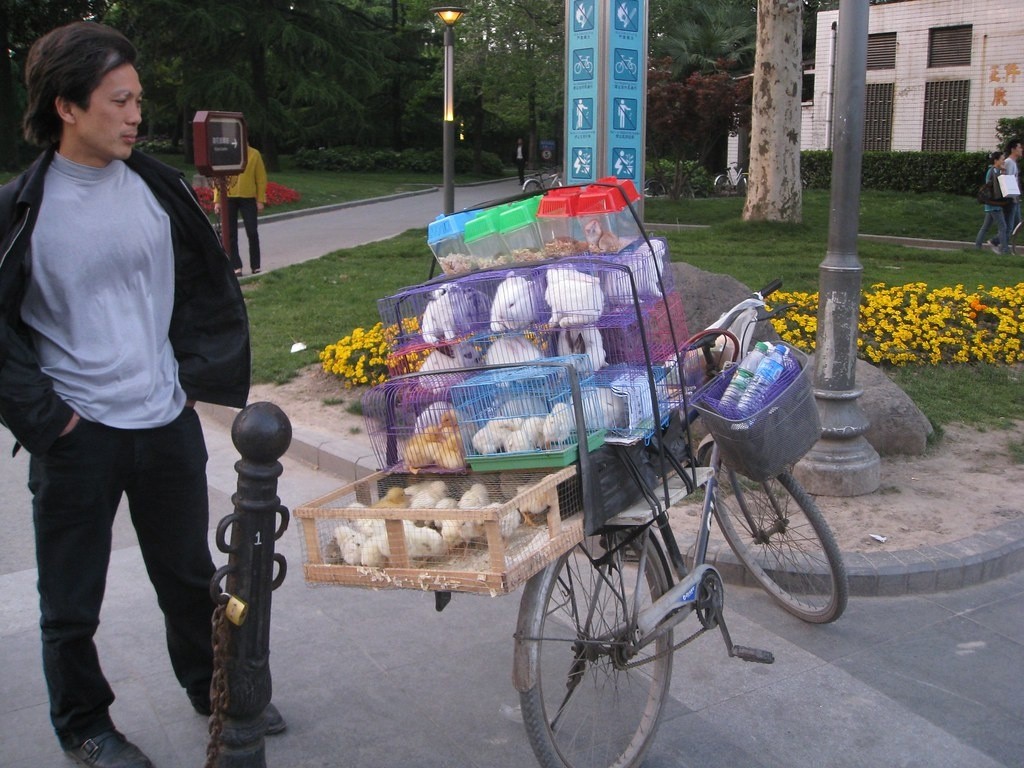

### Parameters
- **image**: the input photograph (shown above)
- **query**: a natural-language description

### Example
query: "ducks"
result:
[333,474,551,568]
[404,403,572,475]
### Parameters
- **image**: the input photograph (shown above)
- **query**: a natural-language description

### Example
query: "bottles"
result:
[736,344,787,419]
[718,341,768,410]
[784,346,791,368]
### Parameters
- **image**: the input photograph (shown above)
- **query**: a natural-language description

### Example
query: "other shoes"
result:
[234,269,242,277]
[252,268,260,274]
[987,240,1001,255]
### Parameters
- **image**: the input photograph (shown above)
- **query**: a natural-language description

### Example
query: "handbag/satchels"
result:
[977,168,1013,206]
[997,174,1021,198]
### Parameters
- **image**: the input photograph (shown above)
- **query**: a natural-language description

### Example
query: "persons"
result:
[212,141,268,276]
[975,138,1023,255]
[0,20,287,768]
[513,138,526,186]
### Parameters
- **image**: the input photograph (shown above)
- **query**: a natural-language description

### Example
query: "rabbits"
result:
[605,239,665,305]
[545,263,604,328]
[557,325,609,371]
[485,271,543,366]
[419,284,493,395]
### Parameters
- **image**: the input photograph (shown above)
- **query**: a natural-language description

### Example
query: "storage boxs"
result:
[426,176,641,275]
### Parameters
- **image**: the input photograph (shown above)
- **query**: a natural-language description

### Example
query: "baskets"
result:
[733,165,745,174]
[692,338,825,482]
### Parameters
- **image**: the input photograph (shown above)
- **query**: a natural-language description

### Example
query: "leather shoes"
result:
[262,703,287,735]
[62,728,155,768]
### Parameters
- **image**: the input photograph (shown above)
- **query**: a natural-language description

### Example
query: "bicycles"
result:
[521,164,562,193]
[714,158,750,193]
[742,171,748,196]
[510,278,849,768]
[643,172,695,200]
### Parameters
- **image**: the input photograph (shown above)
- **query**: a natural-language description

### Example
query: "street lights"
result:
[429,6,471,214]
